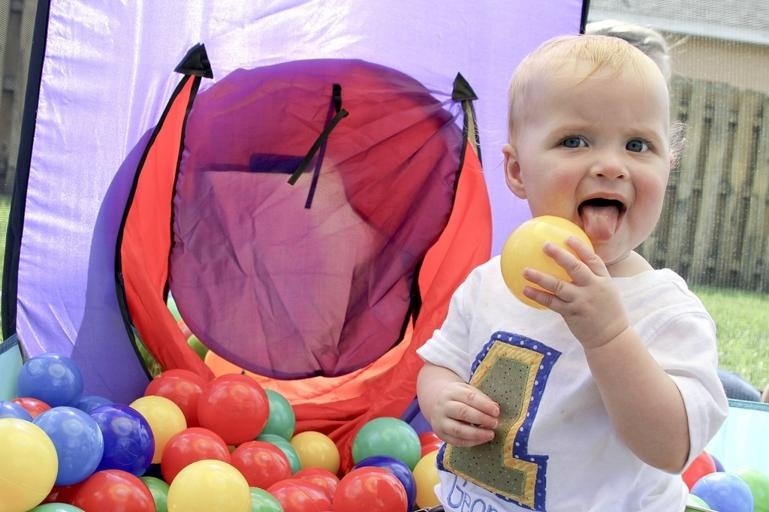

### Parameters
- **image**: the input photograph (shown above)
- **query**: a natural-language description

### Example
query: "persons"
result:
[415,32,734,511]
